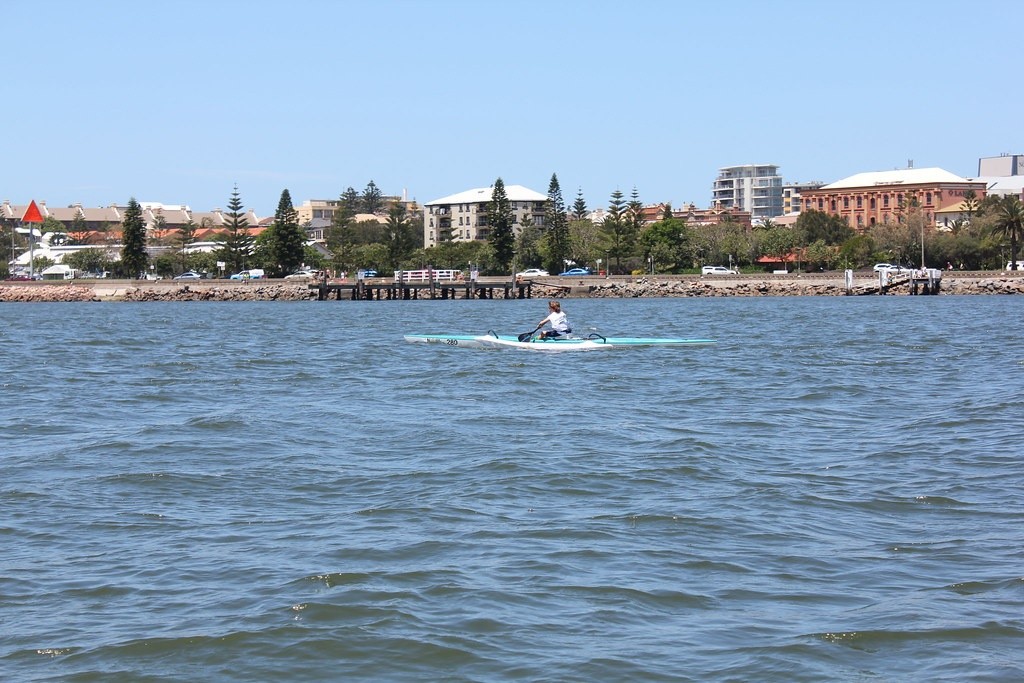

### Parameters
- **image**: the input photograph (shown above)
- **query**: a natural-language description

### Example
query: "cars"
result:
[173,271,203,281]
[231,270,268,280]
[354,268,377,277]
[874,264,917,273]
[1005,261,1024,270]
[558,267,593,276]
[515,268,550,277]
[283,271,317,281]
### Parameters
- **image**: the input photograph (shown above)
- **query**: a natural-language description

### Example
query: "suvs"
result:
[701,265,738,275]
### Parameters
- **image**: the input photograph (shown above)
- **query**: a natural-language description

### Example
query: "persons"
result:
[536,301,573,340]
[452,268,466,281]
[319,267,352,283]
[139,269,148,279]
[909,257,967,280]
[244,273,250,285]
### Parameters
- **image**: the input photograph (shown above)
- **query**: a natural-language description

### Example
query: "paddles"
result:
[518,325,541,342]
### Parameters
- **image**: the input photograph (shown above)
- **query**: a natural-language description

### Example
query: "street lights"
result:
[511,250,519,291]
[605,249,610,279]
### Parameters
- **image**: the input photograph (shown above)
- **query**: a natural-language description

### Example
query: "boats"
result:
[403,328,719,351]
